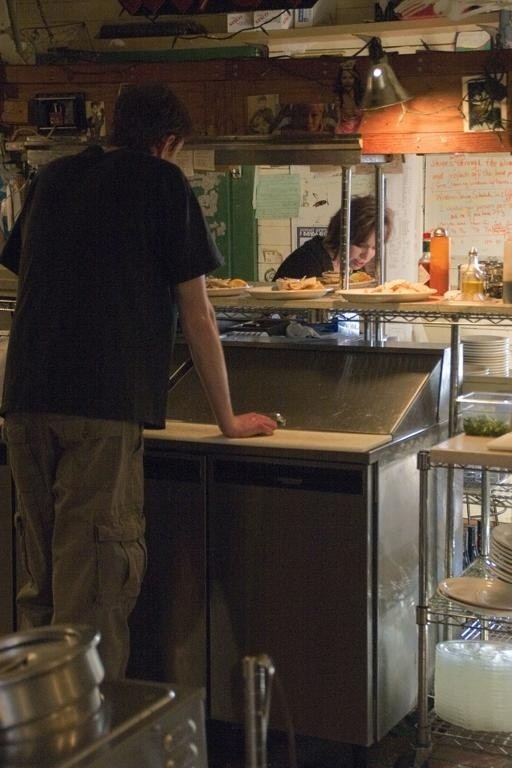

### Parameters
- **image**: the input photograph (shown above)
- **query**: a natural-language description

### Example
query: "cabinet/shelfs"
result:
[416,431,512,756]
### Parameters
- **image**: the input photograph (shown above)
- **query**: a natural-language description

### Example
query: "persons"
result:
[279,104,337,135]
[1,84,277,678]
[272,195,394,281]
[87,102,102,128]
[249,96,278,135]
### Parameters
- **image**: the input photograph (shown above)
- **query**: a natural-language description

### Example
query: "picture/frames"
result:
[462,73,507,133]
[28,92,87,137]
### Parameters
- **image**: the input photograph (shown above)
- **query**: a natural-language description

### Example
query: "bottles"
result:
[431,225,451,292]
[418,232,431,285]
[459,246,485,300]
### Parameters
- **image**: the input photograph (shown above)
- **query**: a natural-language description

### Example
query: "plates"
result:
[459,334,509,380]
[485,522,512,584]
[486,430,512,454]
[431,638,511,734]
[197,274,437,302]
[435,575,512,616]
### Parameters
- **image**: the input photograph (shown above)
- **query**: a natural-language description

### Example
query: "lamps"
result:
[360,36,414,112]
